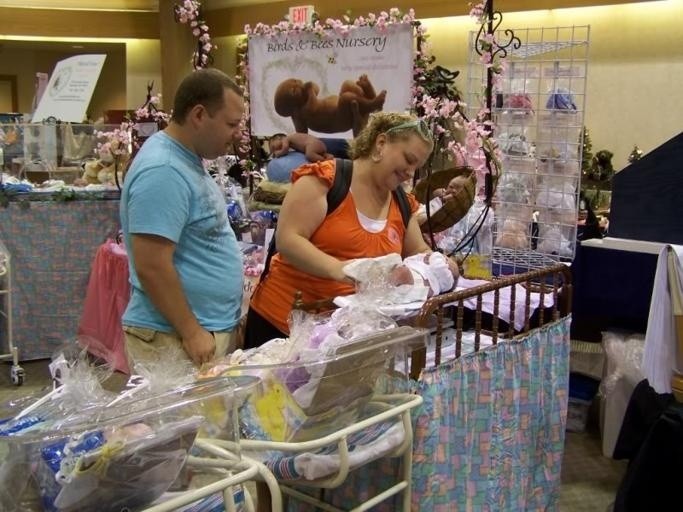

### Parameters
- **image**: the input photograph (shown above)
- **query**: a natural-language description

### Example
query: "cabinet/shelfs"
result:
[0,189,122,362]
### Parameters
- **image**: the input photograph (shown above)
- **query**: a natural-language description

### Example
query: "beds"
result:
[291,264,573,512]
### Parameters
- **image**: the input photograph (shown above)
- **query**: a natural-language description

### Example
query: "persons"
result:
[245,115,460,350]
[270,135,333,166]
[276,76,386,131]
[122,70,244,377]
[416,174,466,226]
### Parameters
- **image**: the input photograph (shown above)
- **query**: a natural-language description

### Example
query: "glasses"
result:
[385,119,433,145]
